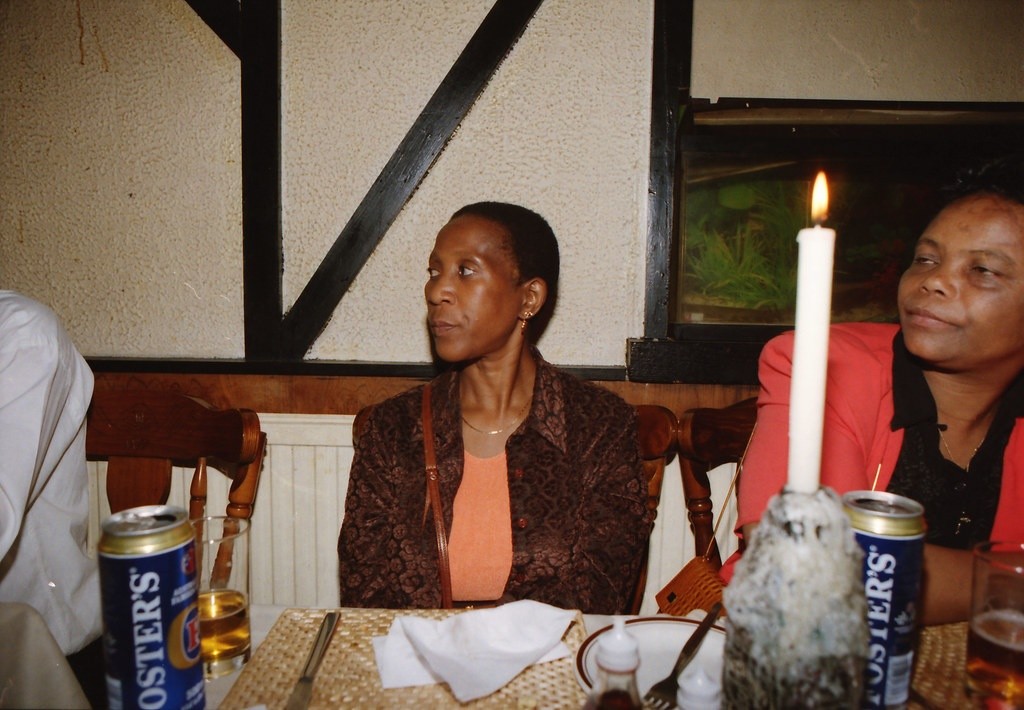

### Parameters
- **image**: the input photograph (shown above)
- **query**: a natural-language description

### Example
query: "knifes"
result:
[281,612,340,710]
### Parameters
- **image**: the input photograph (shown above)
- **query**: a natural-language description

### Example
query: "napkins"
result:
[368,599,580,706]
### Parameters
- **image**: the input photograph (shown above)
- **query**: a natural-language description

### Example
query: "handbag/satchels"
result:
[654,556,727,624]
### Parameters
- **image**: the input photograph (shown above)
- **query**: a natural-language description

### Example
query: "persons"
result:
[1,291,111,709]
[336,201,649,617]
[734,159,1024,627]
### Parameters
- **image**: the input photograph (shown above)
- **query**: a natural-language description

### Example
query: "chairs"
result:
[85,410,266,590]
[627,406,680,616]
[681,397,759,569]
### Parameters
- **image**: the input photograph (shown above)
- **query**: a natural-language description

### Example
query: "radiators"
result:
[86,413,741,627]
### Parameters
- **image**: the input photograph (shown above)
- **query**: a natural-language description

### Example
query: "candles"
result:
[786,172,834,492]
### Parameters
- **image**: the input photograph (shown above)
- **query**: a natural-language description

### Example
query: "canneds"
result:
[842,490,927,710]
[96,504,206,710]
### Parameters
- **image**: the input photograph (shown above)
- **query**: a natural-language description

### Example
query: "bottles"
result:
[582,613,641,710]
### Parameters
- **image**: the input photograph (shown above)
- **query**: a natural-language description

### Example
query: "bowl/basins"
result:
[575,614,725,710]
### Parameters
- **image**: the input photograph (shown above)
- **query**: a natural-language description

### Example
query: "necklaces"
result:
[459,400,533,434]
[939,429,985,472]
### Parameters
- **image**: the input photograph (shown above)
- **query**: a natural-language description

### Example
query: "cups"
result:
[964,540,1024,710]
[188,516,253,680]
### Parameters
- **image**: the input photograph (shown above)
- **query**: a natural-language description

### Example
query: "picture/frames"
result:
[668,94,1024,384]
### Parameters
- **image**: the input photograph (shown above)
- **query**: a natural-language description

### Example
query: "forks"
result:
[640,601,723,709]
